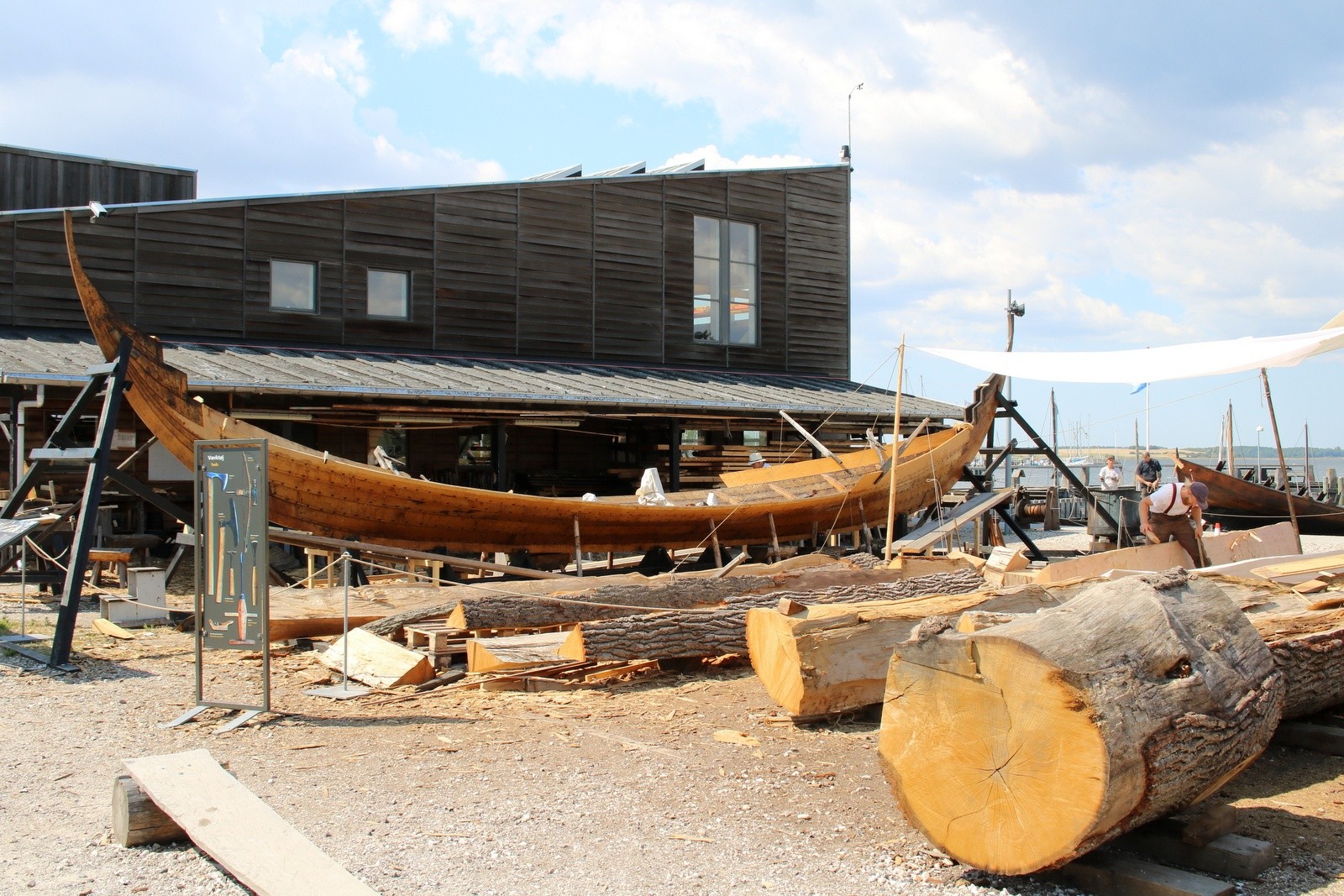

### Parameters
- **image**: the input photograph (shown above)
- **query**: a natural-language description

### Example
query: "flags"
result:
[1129,372,1148,395]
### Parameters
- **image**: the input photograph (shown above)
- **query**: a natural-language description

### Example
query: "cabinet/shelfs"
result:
[99,566,166,629]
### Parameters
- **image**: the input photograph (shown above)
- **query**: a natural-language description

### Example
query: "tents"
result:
[884,326,1343,567]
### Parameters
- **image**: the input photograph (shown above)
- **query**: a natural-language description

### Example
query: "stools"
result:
[89,546,134,589]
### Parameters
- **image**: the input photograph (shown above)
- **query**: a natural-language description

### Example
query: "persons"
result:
[1135,451,1162,499]
[1098,455,1122,491]
[1138,482,1213,569]
[747,452,771,469]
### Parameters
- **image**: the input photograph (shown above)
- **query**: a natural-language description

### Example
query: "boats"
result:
[1175,447,1344,537]
[62,207,1027,557]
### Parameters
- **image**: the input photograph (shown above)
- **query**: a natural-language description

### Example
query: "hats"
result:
[1107,455,1115,460]
[1190,482,1208,510]
[747,453,766,465]
